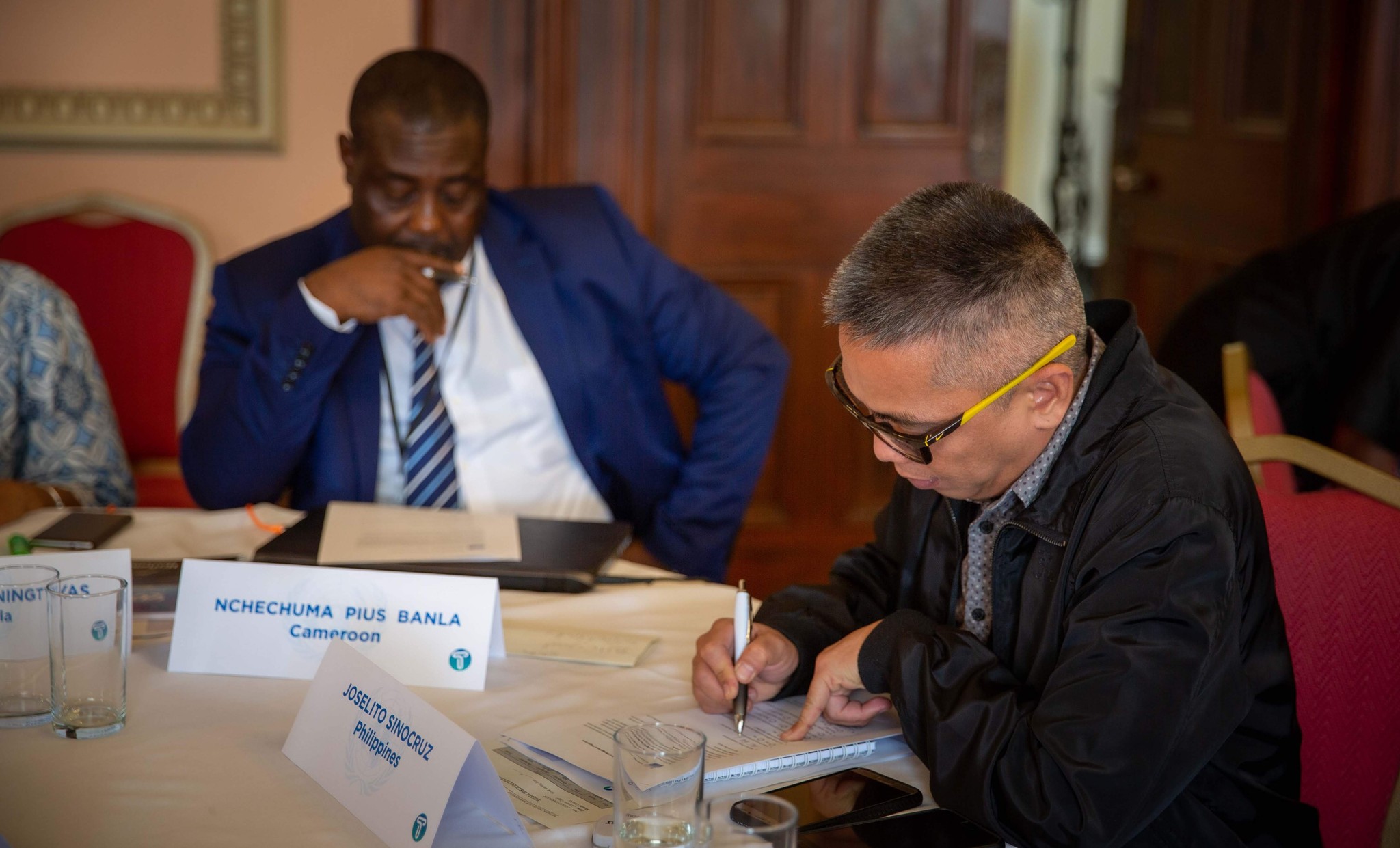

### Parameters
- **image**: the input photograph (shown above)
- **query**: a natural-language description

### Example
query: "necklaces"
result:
[376,239,477,473]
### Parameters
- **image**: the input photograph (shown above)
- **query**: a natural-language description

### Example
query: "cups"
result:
[611,724,706,847]
[0,565,60,728]
[695,792,798,848]
[46,574,127,739]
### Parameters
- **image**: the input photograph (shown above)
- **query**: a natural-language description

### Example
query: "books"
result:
[256,501,634,594]
[702,740,875,783]
[499,696,879,809]
[27,513,132,550]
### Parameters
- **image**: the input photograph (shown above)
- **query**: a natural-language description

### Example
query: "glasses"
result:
[824,335,1077,465]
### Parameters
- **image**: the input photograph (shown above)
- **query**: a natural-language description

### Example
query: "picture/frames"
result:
[0,0,285,153]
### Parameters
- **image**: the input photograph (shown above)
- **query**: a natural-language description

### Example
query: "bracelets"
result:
[35,481,65,507]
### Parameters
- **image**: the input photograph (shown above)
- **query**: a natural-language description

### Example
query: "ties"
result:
[401,279,458,509]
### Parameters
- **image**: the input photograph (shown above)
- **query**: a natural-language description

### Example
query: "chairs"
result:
[1222,343,1400,848]
[0,190,214,510]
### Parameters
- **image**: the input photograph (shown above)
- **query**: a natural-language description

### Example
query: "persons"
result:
[1153,199,1400,492]
[178,46,790,583]
[692,183,1300,848]
[0,261,134,529]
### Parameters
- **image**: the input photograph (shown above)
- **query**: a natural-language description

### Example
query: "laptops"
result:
[249,504,631,594]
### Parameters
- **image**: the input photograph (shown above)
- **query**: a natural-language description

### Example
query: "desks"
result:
[0,529,953,848]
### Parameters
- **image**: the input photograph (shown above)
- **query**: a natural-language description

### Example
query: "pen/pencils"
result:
[10,535,32,554]
[733,579,754,736]
[420,266,466,282]
[101,504,118,515]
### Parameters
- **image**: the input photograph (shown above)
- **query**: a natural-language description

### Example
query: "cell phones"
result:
[28,510,132,549]
[730,767,1006,848]
